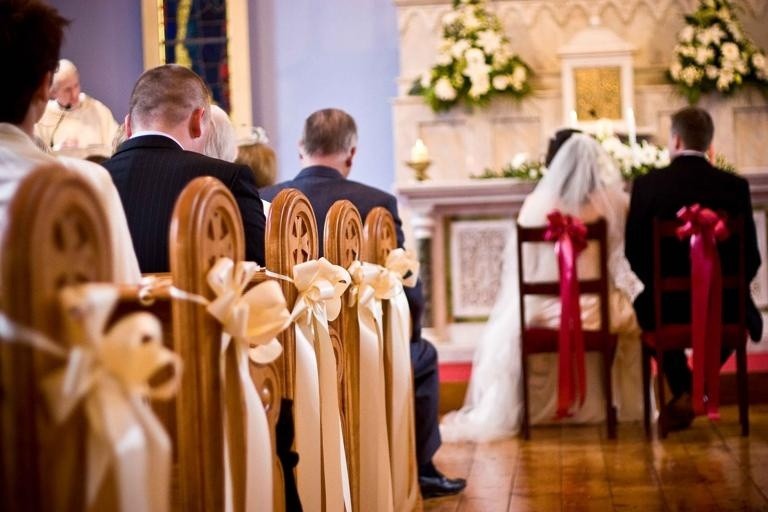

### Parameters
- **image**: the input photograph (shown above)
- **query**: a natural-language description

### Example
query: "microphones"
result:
[50,102,72,147]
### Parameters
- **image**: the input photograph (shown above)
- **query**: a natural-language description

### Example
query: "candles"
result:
[413,139,428,160]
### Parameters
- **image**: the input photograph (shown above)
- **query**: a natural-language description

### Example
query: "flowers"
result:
[665,0,768,108]
[467,118,711,178]
[407,0,546,113]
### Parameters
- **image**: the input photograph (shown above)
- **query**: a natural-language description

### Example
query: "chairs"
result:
[639,213,750,438]
[362,202,423,509]
[321,195,383,508]
[515,217,620,440]
[1,159,126,506]
[128,172,287,511]
[244,184,345,512]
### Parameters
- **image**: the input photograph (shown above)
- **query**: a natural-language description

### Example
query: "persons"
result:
[101,65,267,274]
[625,106,762,433]
[202,104,277,189]
[523,129,656,426]
[0,0,142,284]
[34,59,119,163]
[262,108,467,499]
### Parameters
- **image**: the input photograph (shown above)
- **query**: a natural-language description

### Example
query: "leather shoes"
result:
[416,475,466,499]
